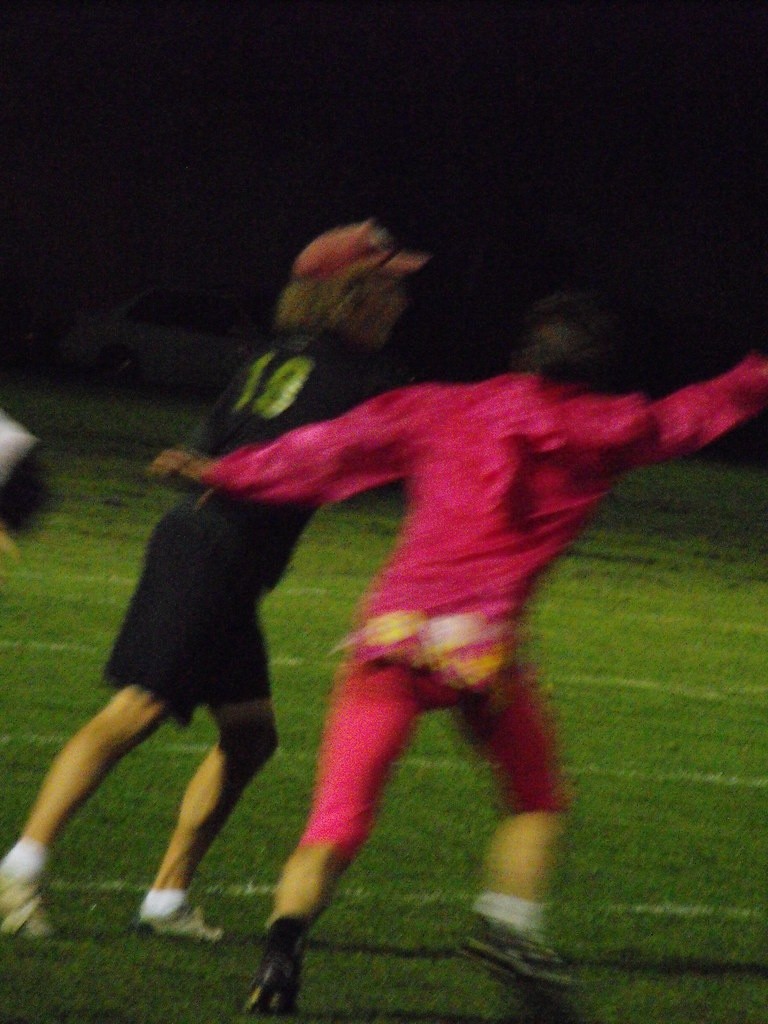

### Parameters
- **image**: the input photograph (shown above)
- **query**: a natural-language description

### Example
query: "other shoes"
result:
[241,948,304,1017]
[135,903,233,949]
[0,873,56,945]
[456,909,590,999]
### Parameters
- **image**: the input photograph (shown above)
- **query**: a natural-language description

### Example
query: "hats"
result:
[291,218,432,279]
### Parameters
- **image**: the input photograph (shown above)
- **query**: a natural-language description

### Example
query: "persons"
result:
[150,295,768,1023]
[0,220,434,949]
